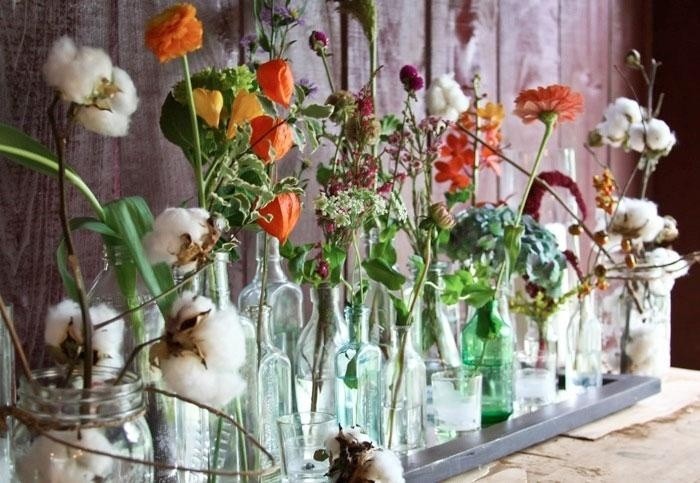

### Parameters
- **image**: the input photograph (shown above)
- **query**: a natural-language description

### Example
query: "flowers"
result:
[149,9,304,312]
[224,0,299,236]
[445,84,584,365]
[331,0,392,357]
[285,22,363,425]
[0,35,407,483]
[391,109,459,376]
[551,167,650,370]
[366,56,423,356]
[436,68,513,369]
[429,40,698,373]
[504,163,612,353]
[0,116,180,383]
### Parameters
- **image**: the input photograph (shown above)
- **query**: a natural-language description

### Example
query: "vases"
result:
[1,227,670,483]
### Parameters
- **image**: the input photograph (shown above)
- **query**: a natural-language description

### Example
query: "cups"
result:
[431,368,483,438]
[276,411,338,483]
[512,349,558,406]
[422,359,451,420]
